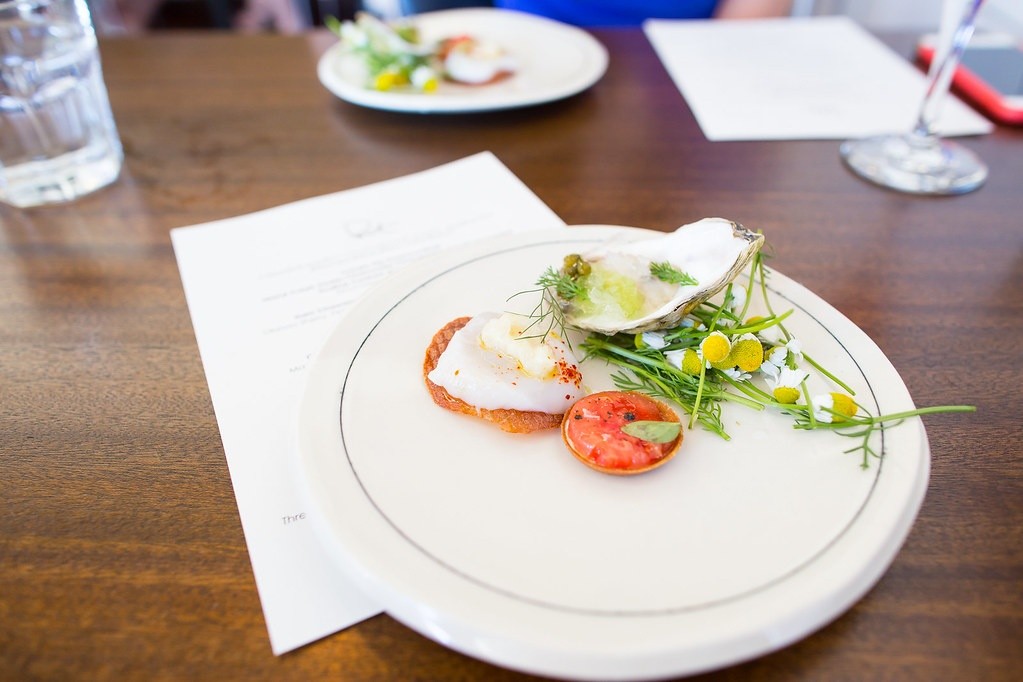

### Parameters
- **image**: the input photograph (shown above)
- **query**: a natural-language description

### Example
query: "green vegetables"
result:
[326,13,440,75]
[505,227,977,468]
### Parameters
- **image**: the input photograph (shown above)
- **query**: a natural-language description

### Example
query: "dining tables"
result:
[0,32,1023,682]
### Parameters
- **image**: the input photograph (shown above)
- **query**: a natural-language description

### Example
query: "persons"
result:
[91,0,793,36]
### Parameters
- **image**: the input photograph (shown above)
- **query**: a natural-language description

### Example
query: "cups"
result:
[0,0,123,208]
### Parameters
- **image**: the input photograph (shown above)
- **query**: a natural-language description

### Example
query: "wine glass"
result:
[839,0,988,195]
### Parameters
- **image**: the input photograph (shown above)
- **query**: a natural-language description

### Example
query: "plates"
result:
[296,225,931,682]
[316,8,610,112]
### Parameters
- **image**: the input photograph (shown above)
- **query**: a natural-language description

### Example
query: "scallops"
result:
[541,217,765,334]
